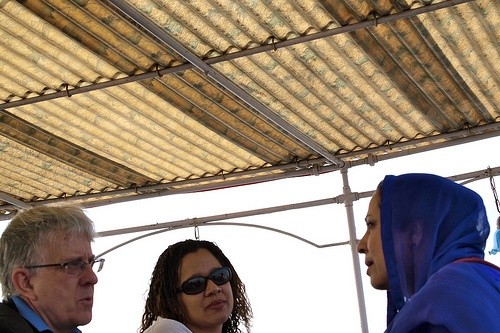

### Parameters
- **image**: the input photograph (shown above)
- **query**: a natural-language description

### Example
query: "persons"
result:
[0,205,106,333]
[139,239,254,333]
[357,173,500,333]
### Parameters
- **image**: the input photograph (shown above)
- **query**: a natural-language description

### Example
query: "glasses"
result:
[21,257,106,275]
[175,266,232,295]
